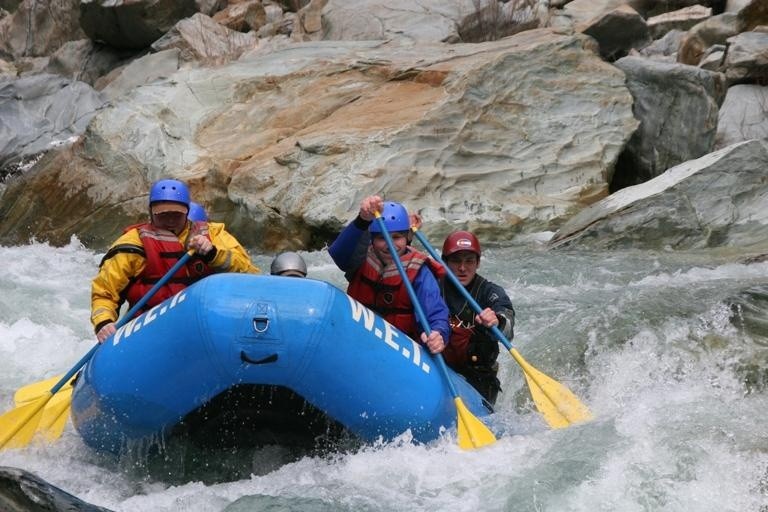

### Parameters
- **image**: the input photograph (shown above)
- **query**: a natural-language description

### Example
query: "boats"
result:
[69,269,507,489]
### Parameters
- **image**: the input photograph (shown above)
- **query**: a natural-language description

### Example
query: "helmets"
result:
[149,180,208,223]
[367,201,409,233]
[270,251,307,280]
[442,230,481,258]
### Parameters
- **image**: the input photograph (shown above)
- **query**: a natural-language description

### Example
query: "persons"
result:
[328,195,449,355]
[407,212,515,407]
[91,178,260,344]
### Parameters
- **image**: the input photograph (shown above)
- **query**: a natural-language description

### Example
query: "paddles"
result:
[373,209,496,448]
[0,245,196,451]
[408,224,594,430]
